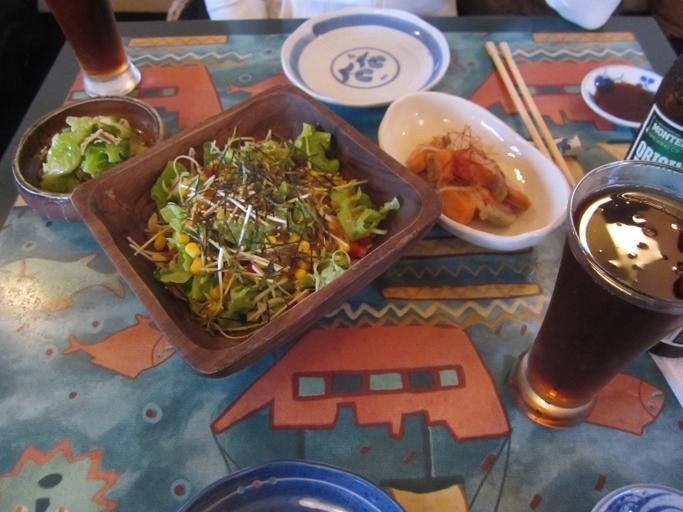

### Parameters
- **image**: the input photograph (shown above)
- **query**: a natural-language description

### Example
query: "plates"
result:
[587,482,682,512]
[373,88,573,254]
[578,63,664,132]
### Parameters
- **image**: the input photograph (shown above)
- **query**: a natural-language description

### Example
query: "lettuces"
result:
[151,120,401,333]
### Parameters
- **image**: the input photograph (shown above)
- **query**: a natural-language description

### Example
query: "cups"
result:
[509,158,682,430]
[42,1,144,102]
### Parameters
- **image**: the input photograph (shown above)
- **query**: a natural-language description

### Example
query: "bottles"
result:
[624,51,681,173]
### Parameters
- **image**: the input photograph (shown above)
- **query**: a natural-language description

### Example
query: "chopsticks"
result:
[483,39,573,200]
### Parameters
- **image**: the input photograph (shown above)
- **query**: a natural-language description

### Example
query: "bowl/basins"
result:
[279,7,453,112]
[11,92,166,231]
[173,459,401,510]
